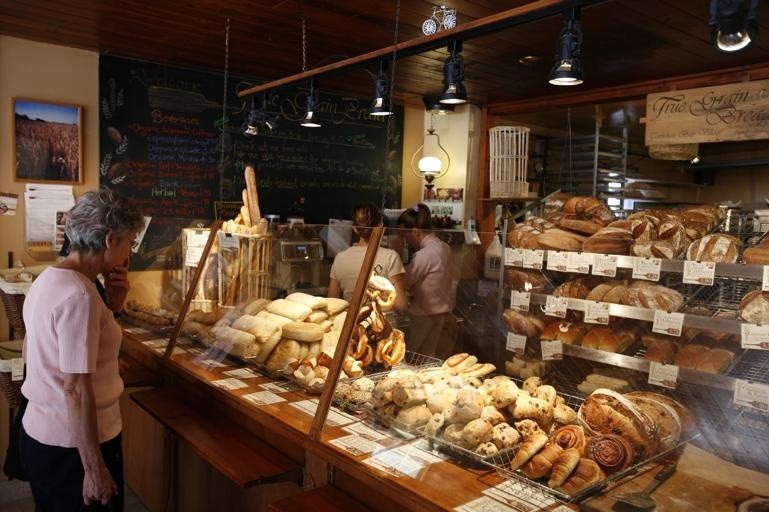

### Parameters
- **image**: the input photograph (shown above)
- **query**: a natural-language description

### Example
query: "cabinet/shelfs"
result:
[543,126,627,212]
[119,219,744,511]
[504,215,769,437]
[624,177,707,213]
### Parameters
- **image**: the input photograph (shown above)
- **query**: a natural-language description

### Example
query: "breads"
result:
[337,353,495,425]
[739,290,769,325]
[121,294,229,342]
[507,308,648,355]
[551,423,633,471]
[642,323,743,377]
[577,387,695,459]
[219,165,270,307]
[500,269,683,311]
[508,192,741,263]
[426,375,576,456]
[744,232,768,265]
[511,434,606,492]
[719,310,736,320]
[687,305,713,318]
[205,273,405,390]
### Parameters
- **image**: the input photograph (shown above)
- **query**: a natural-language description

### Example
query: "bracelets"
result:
[112,310,123,318]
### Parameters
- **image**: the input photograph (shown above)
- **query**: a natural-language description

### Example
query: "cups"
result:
[292,223,302,239]
[277,225,288,238]
[740,214,746,226]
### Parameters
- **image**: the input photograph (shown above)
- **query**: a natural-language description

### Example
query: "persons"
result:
[326,205,409,328]
[397,201,453,362]
[17,186,147,510]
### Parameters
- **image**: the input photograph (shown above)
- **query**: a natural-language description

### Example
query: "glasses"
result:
[127,235,138,249]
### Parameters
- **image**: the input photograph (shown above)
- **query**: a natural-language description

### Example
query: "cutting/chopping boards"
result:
[580,461,769,512]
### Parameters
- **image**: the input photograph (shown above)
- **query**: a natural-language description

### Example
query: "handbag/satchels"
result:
[2,397,38,483]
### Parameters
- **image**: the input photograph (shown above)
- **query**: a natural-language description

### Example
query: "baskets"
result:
[1,364,28,408]
[0,280,27,339]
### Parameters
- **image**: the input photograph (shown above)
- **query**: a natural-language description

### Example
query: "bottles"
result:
[484,236,502,279]
[292,202,297,216]
[300,198,305,217]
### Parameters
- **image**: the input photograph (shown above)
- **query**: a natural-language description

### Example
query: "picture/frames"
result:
[10,96,85,185]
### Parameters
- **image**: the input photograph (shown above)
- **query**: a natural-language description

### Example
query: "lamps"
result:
[548,7,585,85]
[422,94,455,114]
[265,119,278,133]
[301,77,322,129]
[410,114,451,199]
[708,2,757,52]
[244,90,261,138]
[440,42,468,104]
[369,57,395,118]
